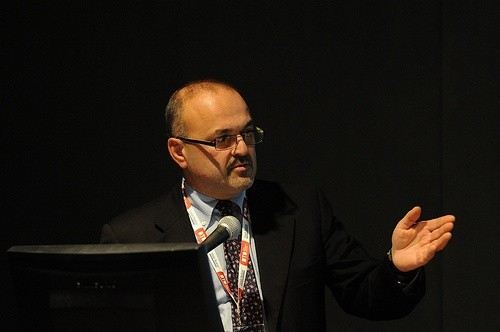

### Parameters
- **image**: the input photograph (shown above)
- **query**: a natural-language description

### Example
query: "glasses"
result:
[176,126,264,152]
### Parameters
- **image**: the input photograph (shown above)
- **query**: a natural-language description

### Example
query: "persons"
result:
[99,76,457,332]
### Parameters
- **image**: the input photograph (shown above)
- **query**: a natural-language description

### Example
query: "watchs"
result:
[386,248,418,286]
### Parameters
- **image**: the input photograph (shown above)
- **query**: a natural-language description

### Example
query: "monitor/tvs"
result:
[5,241,225,332]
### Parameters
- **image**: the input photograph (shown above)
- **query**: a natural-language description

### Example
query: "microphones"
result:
[201,215,242,254]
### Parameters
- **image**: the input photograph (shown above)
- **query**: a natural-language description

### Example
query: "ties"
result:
[213,200,265,332]
